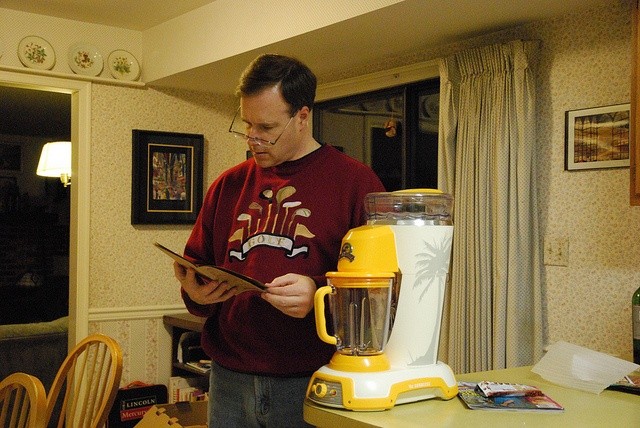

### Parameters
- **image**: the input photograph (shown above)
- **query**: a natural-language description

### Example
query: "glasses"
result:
[228,105,295,147]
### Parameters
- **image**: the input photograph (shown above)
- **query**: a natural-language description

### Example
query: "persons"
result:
[173,52,387,428]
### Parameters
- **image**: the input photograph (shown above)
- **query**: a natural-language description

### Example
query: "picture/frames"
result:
[0,178,16,193]
[0,142,21,172]
[131,128,203,226]
[564,102,630,170]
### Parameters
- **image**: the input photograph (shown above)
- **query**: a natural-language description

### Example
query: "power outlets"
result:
[543,236,569,268]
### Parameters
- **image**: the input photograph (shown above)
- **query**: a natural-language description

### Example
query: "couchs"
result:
[1,315,68,400]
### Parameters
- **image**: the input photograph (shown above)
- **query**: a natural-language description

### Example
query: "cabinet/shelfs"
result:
[162,314,209,401]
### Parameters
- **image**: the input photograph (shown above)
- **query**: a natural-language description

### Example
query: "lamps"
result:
[35,142,73,189]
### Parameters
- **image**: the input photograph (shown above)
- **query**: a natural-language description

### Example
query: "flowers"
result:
[107,49,139,80]
[68,38,103,77]
[18,35,55,69]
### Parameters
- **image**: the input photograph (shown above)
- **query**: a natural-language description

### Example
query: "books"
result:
[455,379,564,413]
[153,242,269,296]
[173,385,208,403]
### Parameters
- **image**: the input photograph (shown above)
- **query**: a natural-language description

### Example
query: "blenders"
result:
[305,188,459,412]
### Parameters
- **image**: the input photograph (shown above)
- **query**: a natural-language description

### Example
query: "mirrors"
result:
[0,73,93,428]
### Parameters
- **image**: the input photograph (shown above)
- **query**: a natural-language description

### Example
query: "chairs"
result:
[0,370,47,428]
[44,333,124,428]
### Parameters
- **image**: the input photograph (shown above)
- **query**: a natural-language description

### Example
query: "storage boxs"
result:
[133,401,209,427]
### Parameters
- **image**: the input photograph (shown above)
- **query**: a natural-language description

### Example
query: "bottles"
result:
[631,285,640,364]
